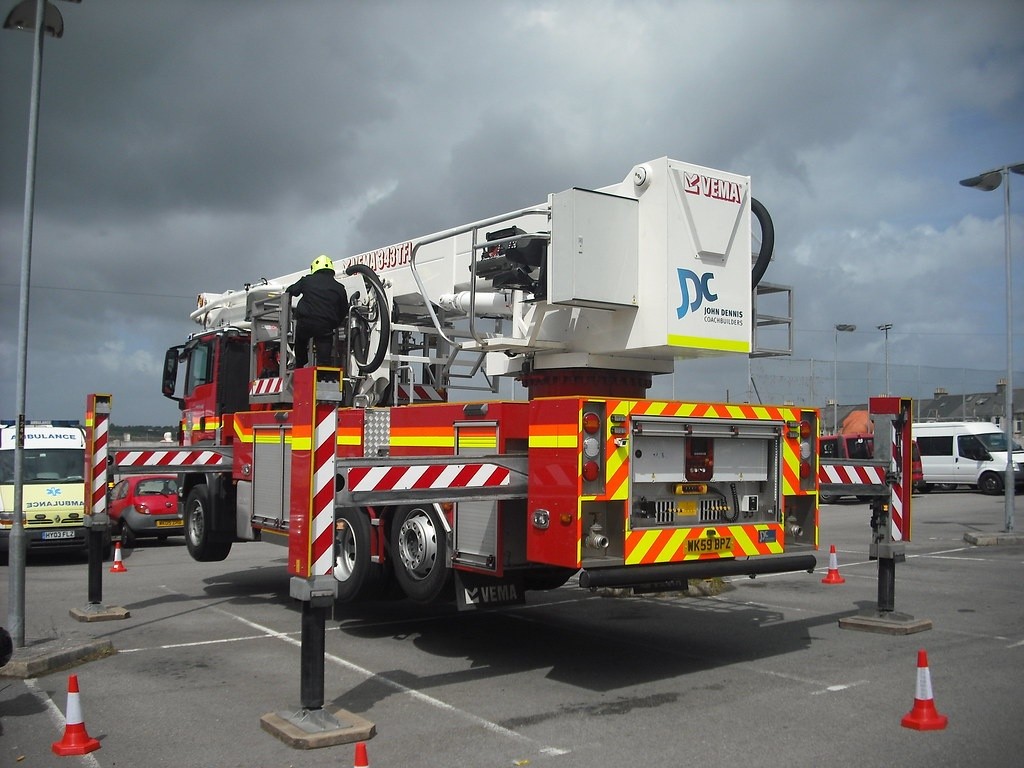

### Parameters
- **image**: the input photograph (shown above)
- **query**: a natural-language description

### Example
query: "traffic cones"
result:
[110,541,128,573]
[353,743,370,768]
[821,545,846,584]
[901,650,948,730]
[52,674,101,756]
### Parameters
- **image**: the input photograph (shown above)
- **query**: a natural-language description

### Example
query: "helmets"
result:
[310,255,335,275]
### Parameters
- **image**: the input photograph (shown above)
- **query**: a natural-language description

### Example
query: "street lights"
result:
[834,324,857,434]
[959,163,1024,533]
[876,323,893,397]
[0,1,63,643]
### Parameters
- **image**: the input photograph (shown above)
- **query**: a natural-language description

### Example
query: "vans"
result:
[892,421,1024,495]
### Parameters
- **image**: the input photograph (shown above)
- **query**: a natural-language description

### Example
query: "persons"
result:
[284,255,347,366]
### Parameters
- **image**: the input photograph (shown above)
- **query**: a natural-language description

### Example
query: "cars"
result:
[109,475,186,549]
[912,440,926,491]
[820,434,874,504]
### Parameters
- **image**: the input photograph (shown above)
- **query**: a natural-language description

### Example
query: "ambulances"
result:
[0,418,112,566]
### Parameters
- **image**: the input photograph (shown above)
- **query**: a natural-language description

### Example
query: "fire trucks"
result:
[70,156,936,751]
